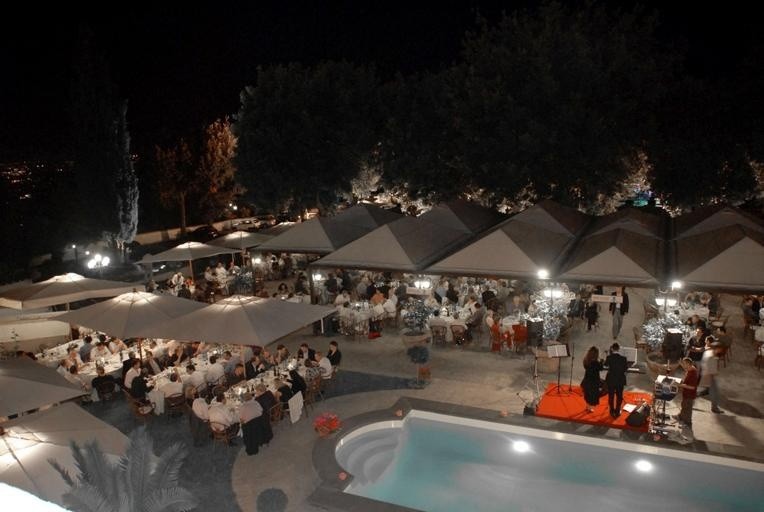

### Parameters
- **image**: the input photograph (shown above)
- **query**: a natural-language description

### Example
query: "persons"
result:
[171,253,310,302]
[327,268,538,340]
[673,295,764,426]
[60,326,342,456]
[580,285,630,416]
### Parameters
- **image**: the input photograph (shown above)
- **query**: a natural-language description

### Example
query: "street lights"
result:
[88,252,112,280]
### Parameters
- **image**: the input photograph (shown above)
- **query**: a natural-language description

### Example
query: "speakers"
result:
[625,401,649,427]
[523,401,537,415]
[526,317,544,347]
[663,328,683,359]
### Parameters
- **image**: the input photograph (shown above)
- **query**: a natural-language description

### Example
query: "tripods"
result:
[516,347,548,406]
